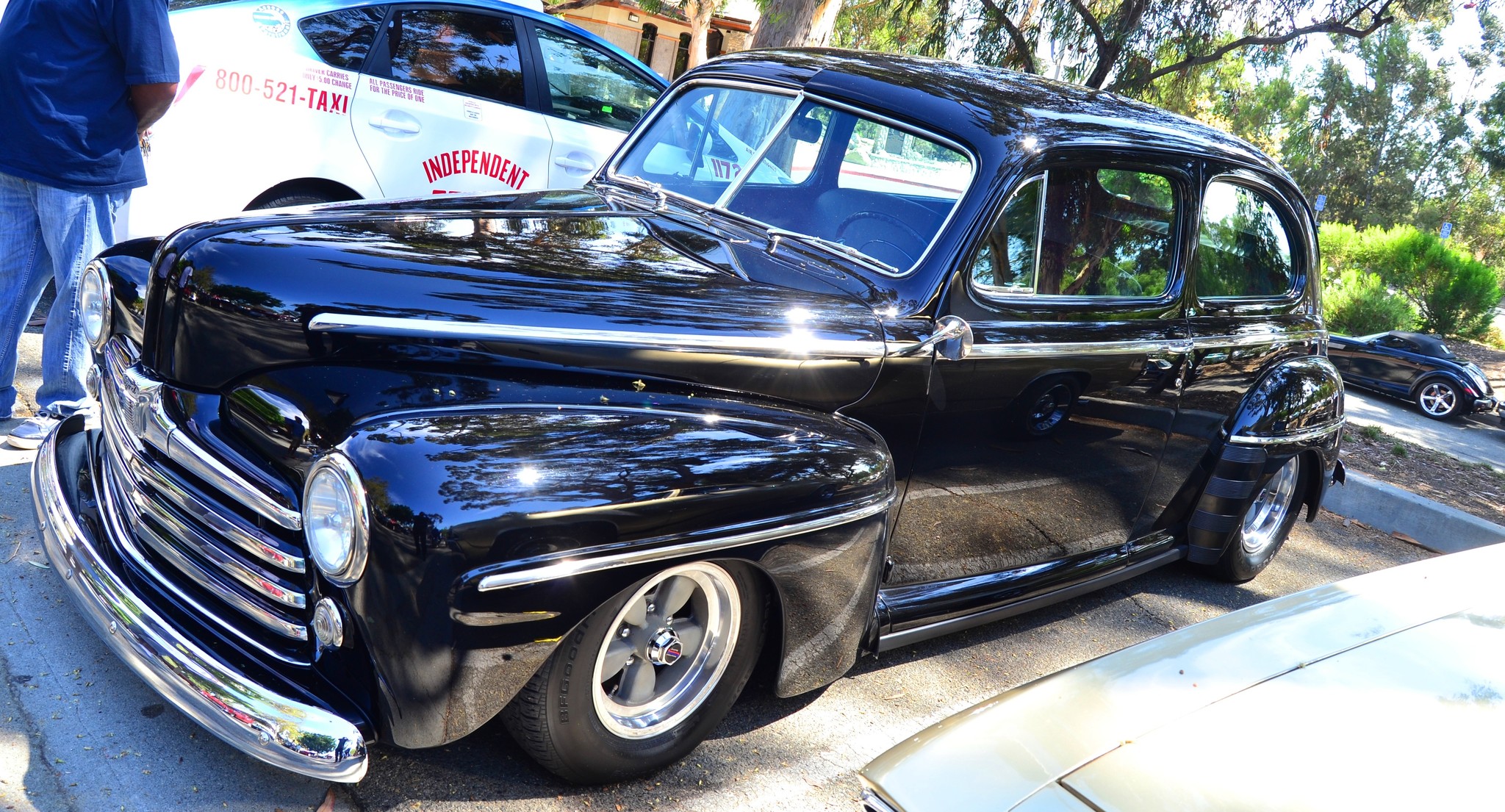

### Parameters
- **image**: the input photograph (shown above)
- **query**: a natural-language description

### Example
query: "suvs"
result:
[28,46,1346,785]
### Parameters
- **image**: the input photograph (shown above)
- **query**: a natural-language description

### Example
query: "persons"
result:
[0,0,181,450]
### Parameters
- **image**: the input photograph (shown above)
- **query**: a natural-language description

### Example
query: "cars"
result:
[1322,331,1505,422]
[109,1,795,254]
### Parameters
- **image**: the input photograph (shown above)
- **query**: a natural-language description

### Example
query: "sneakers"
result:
[7,410,67,450]
